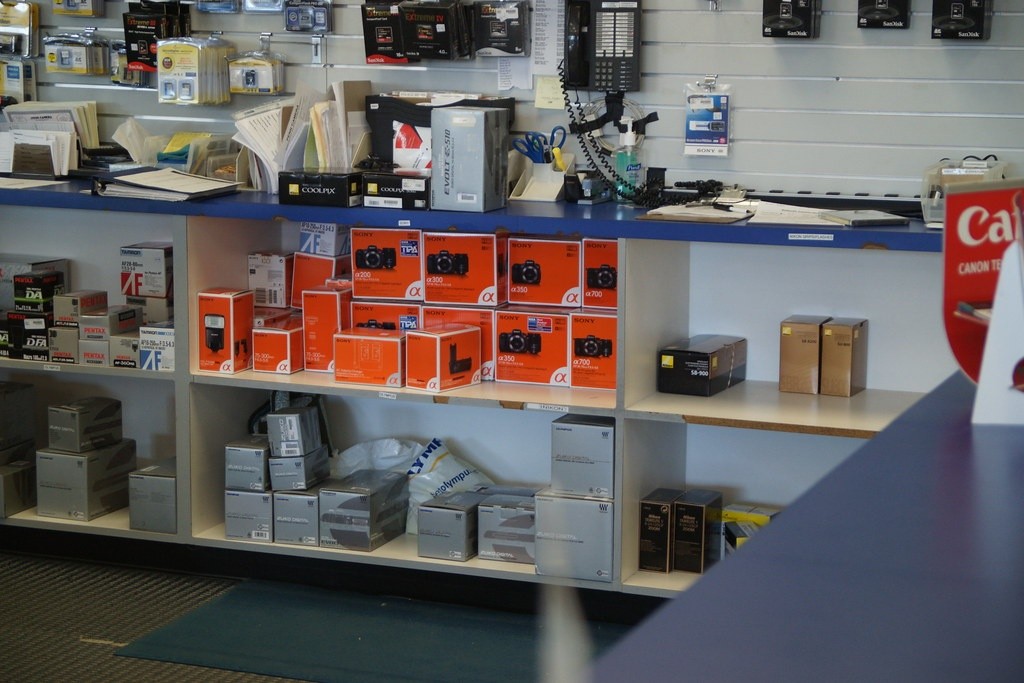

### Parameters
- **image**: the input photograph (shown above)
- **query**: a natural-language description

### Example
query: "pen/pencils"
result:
[713,203,753,215]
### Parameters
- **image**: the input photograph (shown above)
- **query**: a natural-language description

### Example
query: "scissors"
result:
[510,131,545,163]
[531,126,567,162]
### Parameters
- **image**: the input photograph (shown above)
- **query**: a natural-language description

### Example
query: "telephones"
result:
[563,0,641,92]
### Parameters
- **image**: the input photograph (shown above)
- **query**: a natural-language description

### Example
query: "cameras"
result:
[356,246,617,374]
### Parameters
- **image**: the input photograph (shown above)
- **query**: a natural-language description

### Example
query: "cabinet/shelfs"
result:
[0,182,944,629]
[585,370,1024,683]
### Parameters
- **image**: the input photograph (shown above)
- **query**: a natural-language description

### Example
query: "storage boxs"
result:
[550,414,616,499]
[266,407,323,456]
[317,469,410,552]
[920,160,1005,223]
[469,482,542,497]
[121,0,994,214]
[532,484,614,582]
[639,488,687,573]
[223,435,272,492]
[270,477,341,547]
[674,489,723,574]
[749,507,782,525]
[719,504,756,560]
[0,381,177,533]
[267,444,332,491]
[0,241,177,373]
[417,491,488,563]
[198,222,618,392]
[224,489,274,544]
[477,493,535,565]
[724,520,760,561]
[657,311,870,397]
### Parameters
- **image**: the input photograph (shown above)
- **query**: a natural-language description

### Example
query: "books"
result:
[822,210,911,227]
[232,81,373,193]
